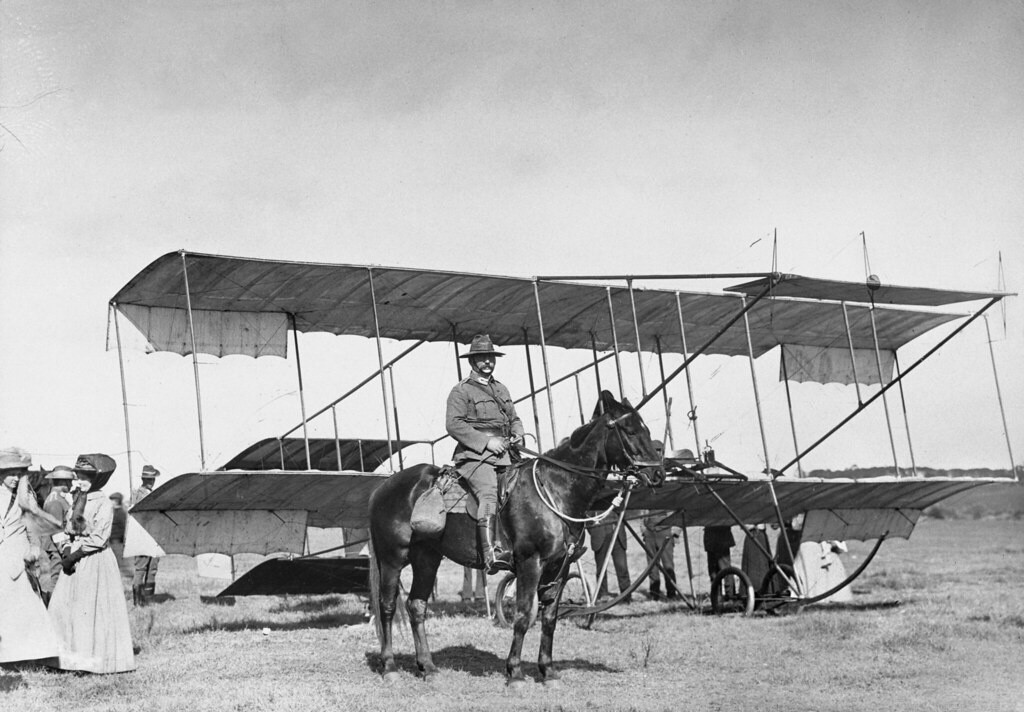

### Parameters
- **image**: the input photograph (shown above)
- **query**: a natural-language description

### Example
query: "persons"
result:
[446,334,526,575]
[586,510,635,605]
[0,446,138,674]
[703,525,853,603]
[131,465,160,608]
[640,509,682,601]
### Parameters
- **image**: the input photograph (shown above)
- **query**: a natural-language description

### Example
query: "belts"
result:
[472,425,510,437]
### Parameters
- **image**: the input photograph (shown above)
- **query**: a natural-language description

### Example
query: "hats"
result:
[0,449,32,469]
[45,465,73,480]
[70,456,96,471]
[138,465,160,478]
[458,334,506,357]
[653,440,663,450]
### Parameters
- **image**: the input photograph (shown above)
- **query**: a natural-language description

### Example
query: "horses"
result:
[367,389,668,689]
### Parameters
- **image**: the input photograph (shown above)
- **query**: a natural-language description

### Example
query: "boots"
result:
[478,514,511,575]
[133,582,155,608]
[647,580,660,601]
[665,577,676,599]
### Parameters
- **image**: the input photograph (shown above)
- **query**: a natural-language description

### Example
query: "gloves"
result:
[62,547,85,576]
